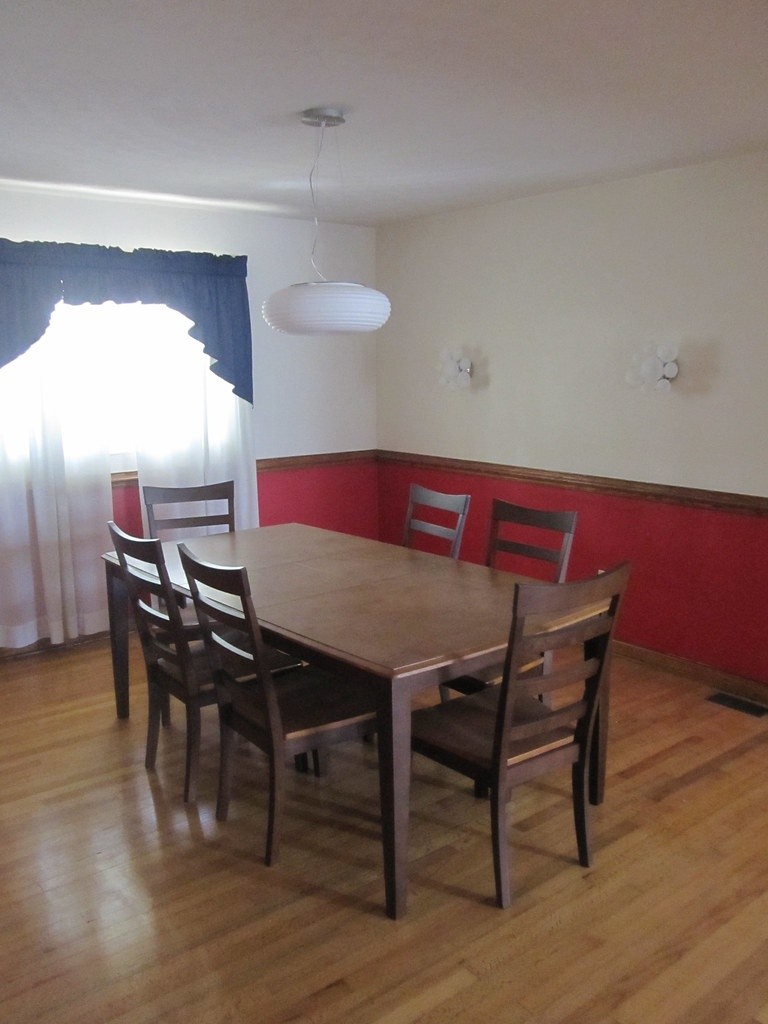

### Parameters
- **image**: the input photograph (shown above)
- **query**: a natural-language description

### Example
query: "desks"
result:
[99,522,611,922]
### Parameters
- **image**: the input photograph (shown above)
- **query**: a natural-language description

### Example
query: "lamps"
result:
[440,358,473,387]
[262,108,392,334]
[640,344,681,393]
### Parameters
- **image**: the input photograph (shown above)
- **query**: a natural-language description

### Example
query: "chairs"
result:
[107,481,635,908]
